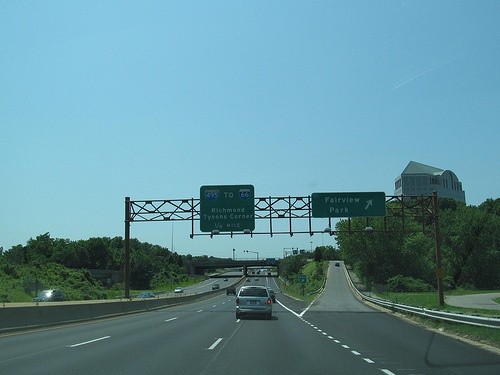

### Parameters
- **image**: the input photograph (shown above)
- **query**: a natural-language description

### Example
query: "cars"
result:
[212,283,219,290]
[174,287,183,293]
[138,292,156,298]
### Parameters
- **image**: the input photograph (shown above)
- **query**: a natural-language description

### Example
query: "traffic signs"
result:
[312,192,386,218]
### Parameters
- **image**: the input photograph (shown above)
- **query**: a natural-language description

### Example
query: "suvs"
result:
[32,289,64,302]
[227,286,236,295]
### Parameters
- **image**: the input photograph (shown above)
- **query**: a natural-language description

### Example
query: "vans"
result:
[234,285,276,319]
